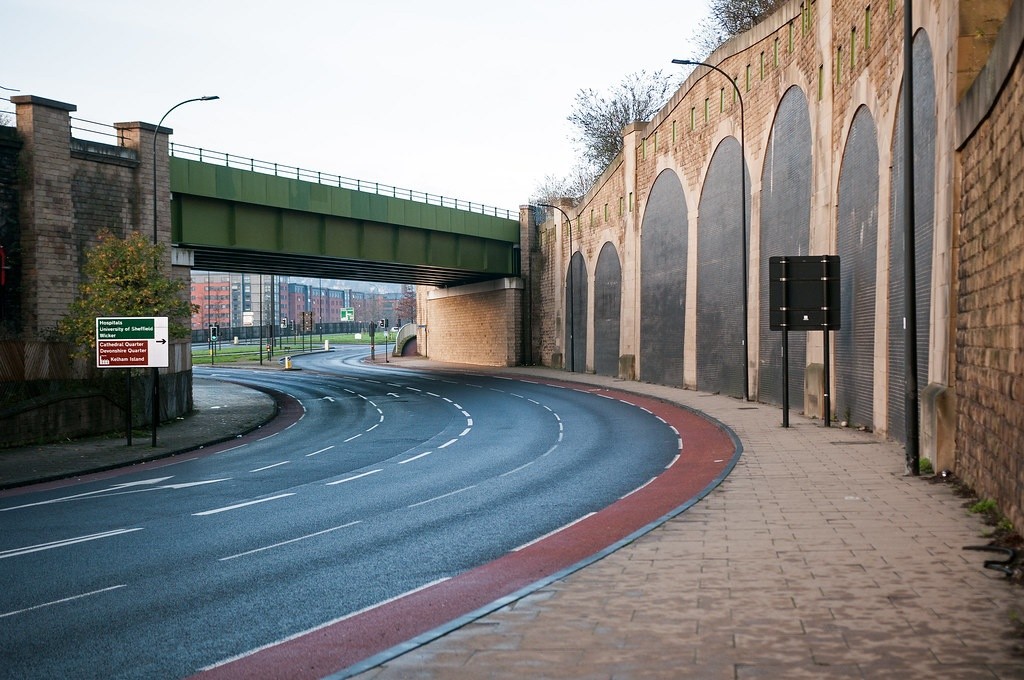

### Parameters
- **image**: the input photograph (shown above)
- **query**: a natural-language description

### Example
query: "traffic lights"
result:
[210,327,219,342]
[283,318,288,329]
[214,323,219,329]
[379,318,386,329]
[290,321,295,330]
[386,320,390,328]
[370,321,377,337]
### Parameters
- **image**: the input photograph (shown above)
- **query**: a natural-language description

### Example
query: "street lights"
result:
[146,95,222,441]
[537,203,578,374]
[670,58,757,403]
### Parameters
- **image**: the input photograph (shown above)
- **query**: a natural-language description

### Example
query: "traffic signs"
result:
[96,316,170,368]
